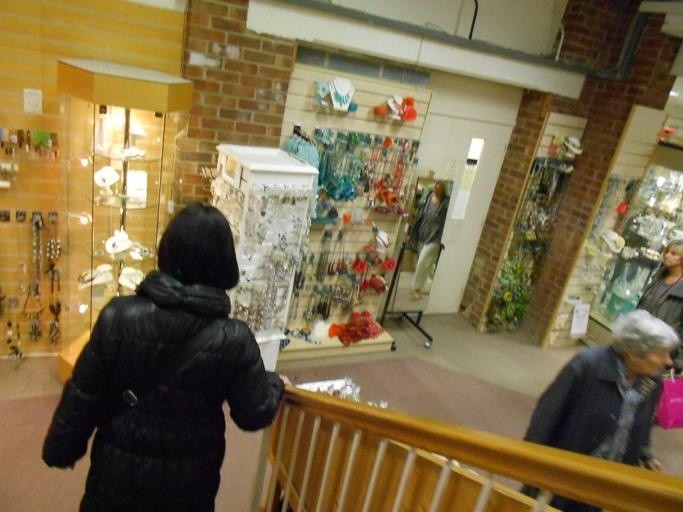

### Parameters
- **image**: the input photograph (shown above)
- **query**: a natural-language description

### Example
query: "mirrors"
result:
[384,176,454,315]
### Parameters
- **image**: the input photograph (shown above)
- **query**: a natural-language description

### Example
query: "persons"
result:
[41,202,293,512]
[406,178,451,300]
[632,238,683,428]
[516,308,680,512]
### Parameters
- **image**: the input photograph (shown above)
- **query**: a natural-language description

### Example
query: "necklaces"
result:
[332,80,352,106]
[392,96,405,116]
[0,129,151,359]
[210,125,420,333]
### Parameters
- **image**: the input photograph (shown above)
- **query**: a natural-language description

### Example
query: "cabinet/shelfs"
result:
[53,62,194,385]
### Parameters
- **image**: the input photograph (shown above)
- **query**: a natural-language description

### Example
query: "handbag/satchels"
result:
[655,365,683,429]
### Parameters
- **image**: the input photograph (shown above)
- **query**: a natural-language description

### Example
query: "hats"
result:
[563,136,583,156]
